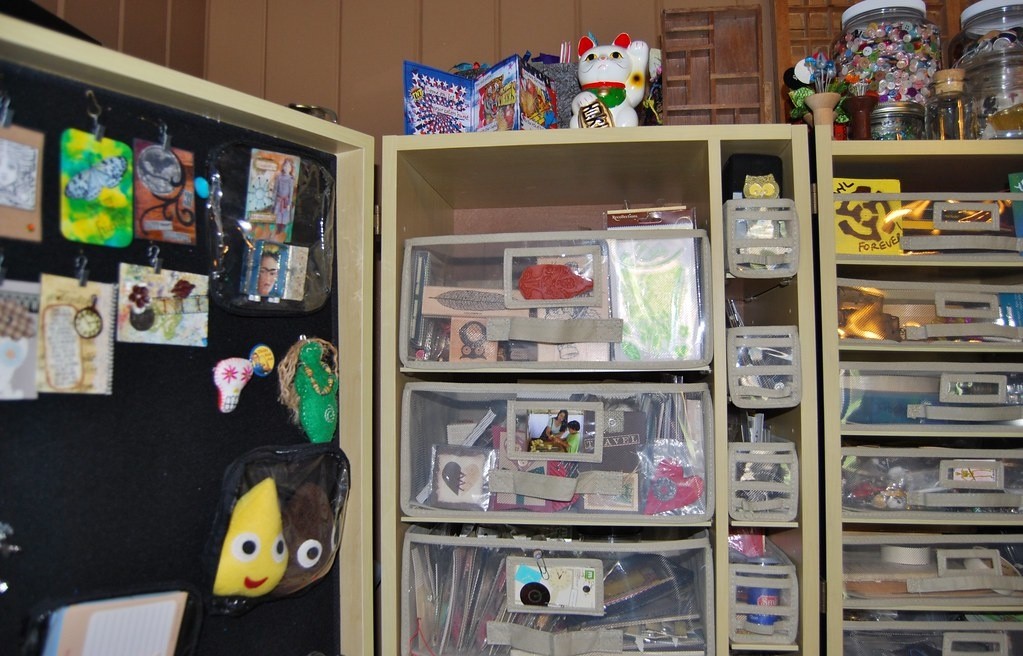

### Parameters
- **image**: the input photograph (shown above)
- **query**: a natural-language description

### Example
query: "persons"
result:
[273,158,295,234]
[539,410,580,453]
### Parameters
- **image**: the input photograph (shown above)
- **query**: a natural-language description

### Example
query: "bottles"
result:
[948,0,1023,139]
[926,67,980,139]
[827,0,942,139]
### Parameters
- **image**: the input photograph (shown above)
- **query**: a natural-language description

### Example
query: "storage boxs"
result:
[389,222,715,655]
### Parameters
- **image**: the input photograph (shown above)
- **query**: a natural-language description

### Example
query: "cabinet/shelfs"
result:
[374,121,821,656]
[813,121,1022,656]
